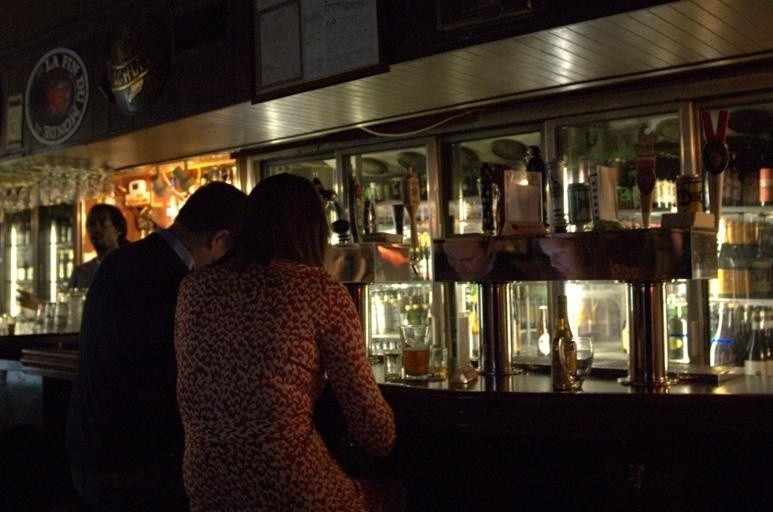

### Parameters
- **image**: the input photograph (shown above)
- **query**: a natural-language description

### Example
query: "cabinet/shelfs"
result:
[0,0,772,384]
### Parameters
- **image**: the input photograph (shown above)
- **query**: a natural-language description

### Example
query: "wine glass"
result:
[555,335,596,393]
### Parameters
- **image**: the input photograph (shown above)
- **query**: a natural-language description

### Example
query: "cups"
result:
[397,324,430,379]
[383,349,403,383]
[428,346,448,381]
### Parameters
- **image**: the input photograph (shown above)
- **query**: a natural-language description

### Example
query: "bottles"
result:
[468,286,481,369]
[666,301,687,361]
[709,139,773,394]
[536,304,551,357]
[548,294,577,394]
[370,289,430,336]
[0,283,84,336]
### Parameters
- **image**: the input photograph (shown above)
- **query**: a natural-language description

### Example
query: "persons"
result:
[14,201,133,315]
[65,176,249,512]
[171,172,398,511]
